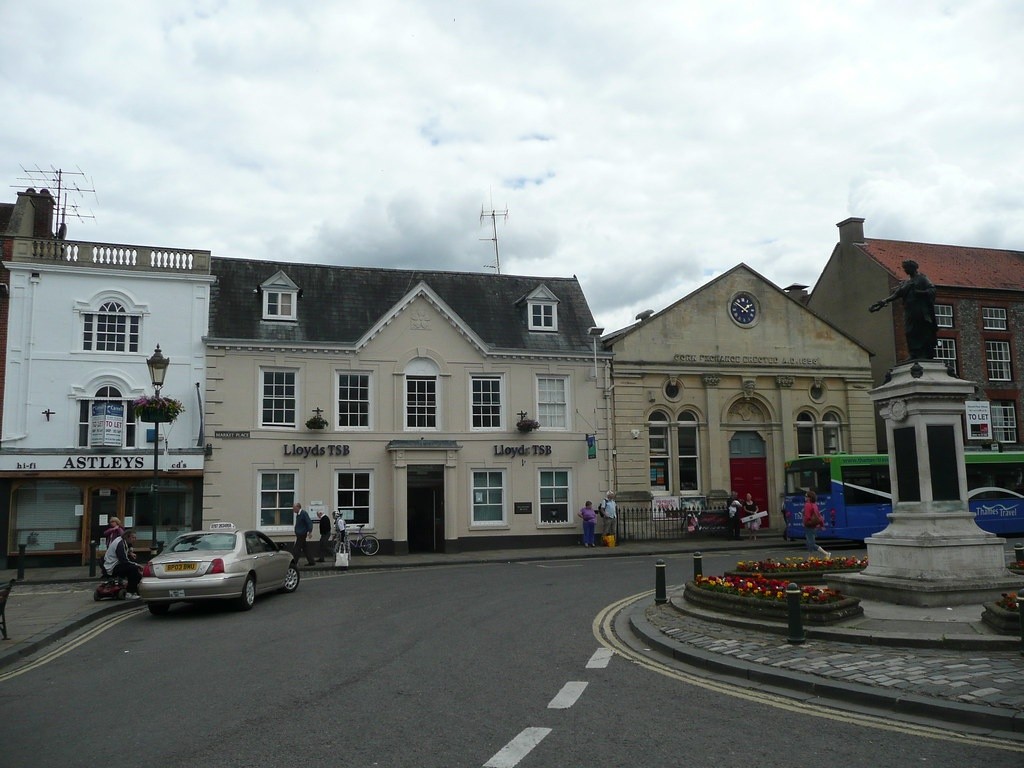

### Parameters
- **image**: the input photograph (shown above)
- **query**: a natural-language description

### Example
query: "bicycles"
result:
[330,524,379,557]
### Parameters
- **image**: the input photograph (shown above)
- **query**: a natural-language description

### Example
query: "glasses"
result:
[805,496,810,498]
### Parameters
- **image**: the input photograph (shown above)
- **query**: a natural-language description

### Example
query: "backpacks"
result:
[598,498,608,518]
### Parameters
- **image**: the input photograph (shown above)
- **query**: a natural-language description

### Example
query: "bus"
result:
[782,448,1023,546]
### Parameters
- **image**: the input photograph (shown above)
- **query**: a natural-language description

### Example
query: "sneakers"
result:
[125,593,140,600]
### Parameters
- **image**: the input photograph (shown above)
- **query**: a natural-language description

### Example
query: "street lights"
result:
[145,342,171,563]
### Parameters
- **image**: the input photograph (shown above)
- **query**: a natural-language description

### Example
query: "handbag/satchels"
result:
[745,512,762,532]
[602,534,614,547]
[802,503,821,528]
[334,543,348,567]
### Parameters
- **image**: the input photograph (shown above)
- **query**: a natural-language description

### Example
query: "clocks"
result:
[731,295,756,324]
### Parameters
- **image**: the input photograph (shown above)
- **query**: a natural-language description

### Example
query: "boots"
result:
[817,546,831,561]
[806,552,814,562]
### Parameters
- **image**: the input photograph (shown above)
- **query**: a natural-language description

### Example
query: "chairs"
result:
[0,578,15,640]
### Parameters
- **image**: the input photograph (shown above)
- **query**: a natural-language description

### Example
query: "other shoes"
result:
[782,533,788,541]
[304,563,316,567]
[316,558,325,563]
[584,544,595,548]
[728,536,744,541]
[790,538,797,541]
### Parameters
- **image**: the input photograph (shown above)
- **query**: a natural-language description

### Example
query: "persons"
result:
[803,491,831,558]
[882,260,939,359]
[291,502,316,566]
[601,490,616,535]
[104,529,143,599]
[103,517,125,547]
[316,511,332,562]
[577,500,597,547]
[727,491,758,541]
[332,511,345,553]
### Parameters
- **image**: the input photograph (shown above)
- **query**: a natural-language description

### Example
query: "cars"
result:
[138,521,306,613]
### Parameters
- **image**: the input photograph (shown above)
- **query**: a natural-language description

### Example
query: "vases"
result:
[312,424,325,429]
[522,427,533,432]
[141,412,173,422]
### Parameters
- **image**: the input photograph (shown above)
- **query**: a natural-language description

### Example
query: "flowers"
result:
[737,554,869,571]
[516,420,540,431]
[683,578,841,603]
[995,591,1019,611]
[305,416,329,429]
[1010,561,1024,569]
[133,396,186,423]
